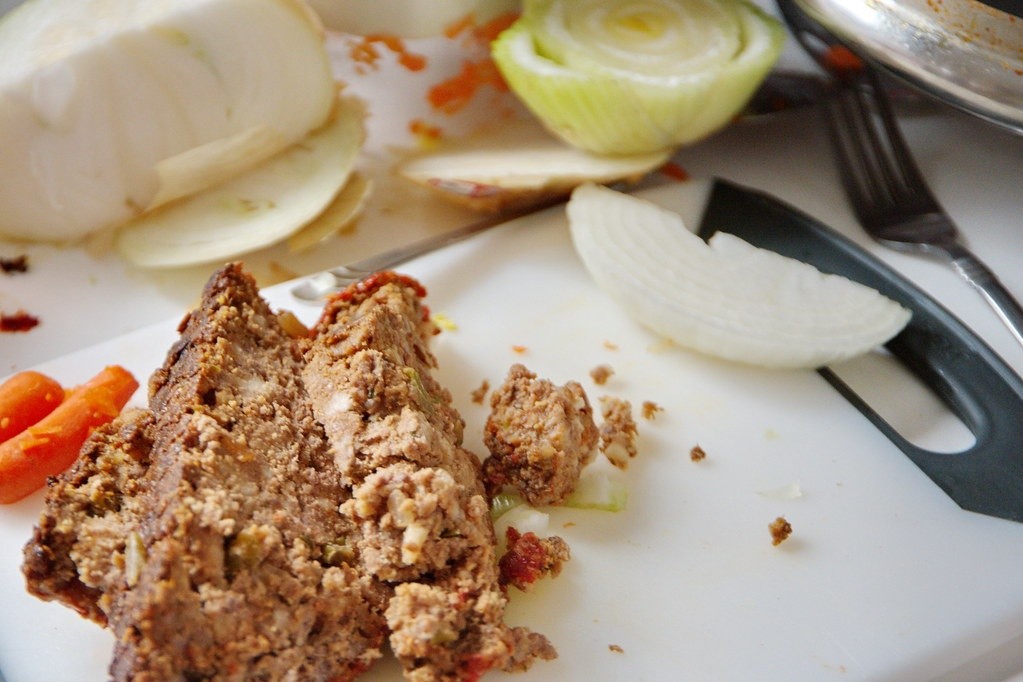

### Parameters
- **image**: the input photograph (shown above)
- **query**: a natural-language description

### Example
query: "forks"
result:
[821,69,1022,347]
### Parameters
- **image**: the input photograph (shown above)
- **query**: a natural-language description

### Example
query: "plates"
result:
[0,115,1023,682]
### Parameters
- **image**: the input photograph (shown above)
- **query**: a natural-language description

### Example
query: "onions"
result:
[397,137,677,218]
[488,0,778,158]
[0,0,372,268]
[566,179,914,370]
